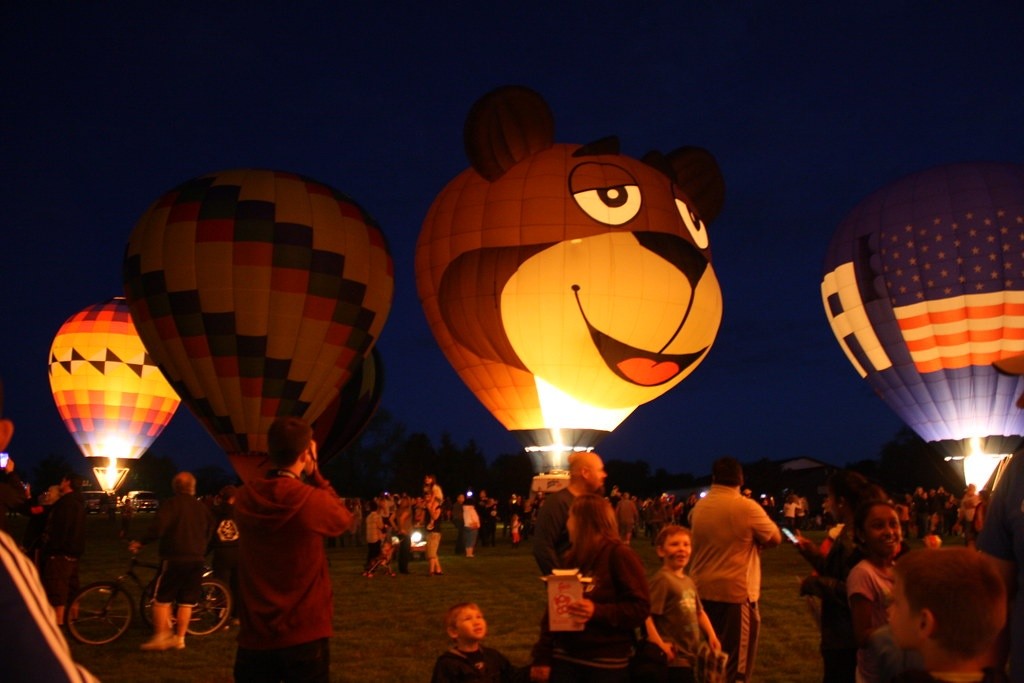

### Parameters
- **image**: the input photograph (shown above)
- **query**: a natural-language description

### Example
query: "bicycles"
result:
[64,538,233,646]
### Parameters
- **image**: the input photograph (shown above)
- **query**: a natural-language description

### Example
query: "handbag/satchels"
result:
[632,640,667,683]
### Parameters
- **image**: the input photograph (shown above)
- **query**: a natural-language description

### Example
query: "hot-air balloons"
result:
[415,88,726,538]
[48,297,181,496]
[821,163,1024,498]
[125,172,393,488]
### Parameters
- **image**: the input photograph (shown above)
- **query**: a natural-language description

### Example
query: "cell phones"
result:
[0,451,9,468]
[782,528,799,544]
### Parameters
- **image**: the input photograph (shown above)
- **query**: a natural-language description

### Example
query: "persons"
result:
[531,451,784,683]
[233,417,353,683]
[0,456,101,683]
[107,499,132,538]
[130,471,215,650]
[781,441,1024,683]
[204,485,239,630]
[324,472,545,578]
[430,601,551,683]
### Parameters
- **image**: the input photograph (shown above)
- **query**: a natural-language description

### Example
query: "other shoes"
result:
[141,635,185,650]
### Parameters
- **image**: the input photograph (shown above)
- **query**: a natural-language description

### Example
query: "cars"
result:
[80,490,159,512]
